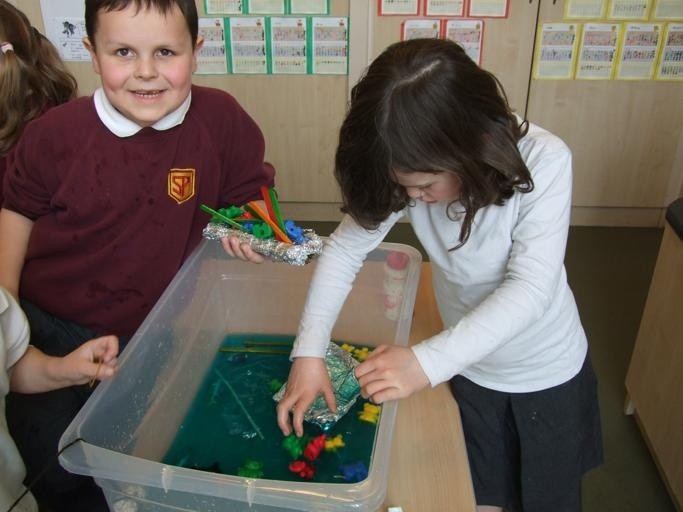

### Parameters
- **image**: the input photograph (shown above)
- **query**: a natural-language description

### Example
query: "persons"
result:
[1,0,79,164]
[276,38,598,512]
[1,286,119,511]
[0,2,278,511]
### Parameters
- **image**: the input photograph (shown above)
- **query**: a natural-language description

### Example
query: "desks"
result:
[128,255,476,512]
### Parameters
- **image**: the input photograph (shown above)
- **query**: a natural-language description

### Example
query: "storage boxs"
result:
[54,233,424,512]
